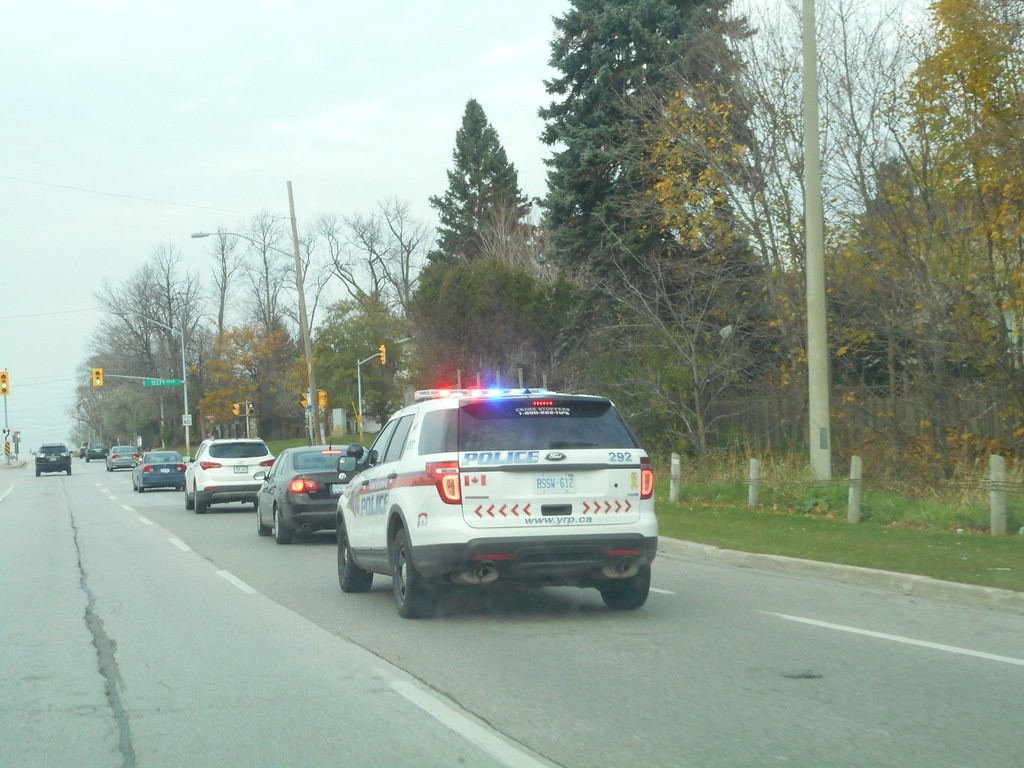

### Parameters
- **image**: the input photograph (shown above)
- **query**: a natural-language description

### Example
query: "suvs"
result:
[79,441,109,462]
[182,435,276,514]
[32,443,73,476]
[335,389,658,619]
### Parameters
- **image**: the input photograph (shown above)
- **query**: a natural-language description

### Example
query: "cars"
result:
[254,444,373,544]
[105,446,142,471]
[131,451,186,493]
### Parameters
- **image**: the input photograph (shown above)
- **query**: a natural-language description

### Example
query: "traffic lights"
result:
[317,390,328,406]
[232,403,240,416]
[299,392,308,408]
[378,344,386,364]
[0,370,10,395]
[93,368,103,387]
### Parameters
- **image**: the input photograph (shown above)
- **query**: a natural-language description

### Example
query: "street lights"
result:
[191,232,323,444]
[113,311,190,455]
